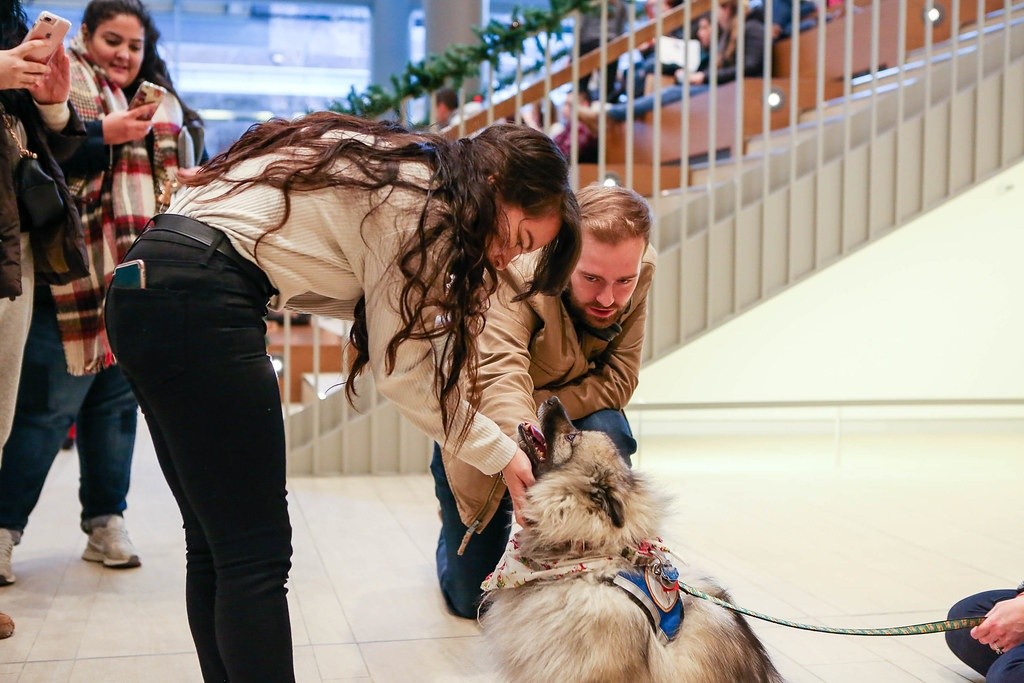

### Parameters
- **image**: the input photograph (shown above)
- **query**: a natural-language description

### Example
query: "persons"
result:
[946,584,1024,683]
[257,0,834,328]
[103,110,583,683]
[0,0,213,641]
[429,183,657,620]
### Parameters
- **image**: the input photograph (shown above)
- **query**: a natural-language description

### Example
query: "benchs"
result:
[580,164,692,196]
[611,77,853,165]
[775,0,1003,77]
[266,324,358,404]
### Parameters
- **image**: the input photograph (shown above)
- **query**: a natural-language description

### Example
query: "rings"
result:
[993,642,1003,655]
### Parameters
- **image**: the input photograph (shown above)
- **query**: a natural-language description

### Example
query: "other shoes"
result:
[573,106,613,137]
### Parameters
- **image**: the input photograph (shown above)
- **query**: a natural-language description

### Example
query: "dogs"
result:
[473,396,791,683]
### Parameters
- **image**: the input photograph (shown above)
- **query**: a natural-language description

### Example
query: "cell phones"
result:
[127,81,167,120]
[21,11,71,67]
[114,259,145,289]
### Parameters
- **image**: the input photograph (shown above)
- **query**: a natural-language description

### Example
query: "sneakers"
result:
[79,512,141,570]
[0,524,24,588]
[0,612,16,641]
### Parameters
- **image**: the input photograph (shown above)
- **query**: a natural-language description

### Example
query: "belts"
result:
[140,213,247,261]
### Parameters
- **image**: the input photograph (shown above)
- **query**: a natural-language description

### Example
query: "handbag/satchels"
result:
[14,154,66,233]
